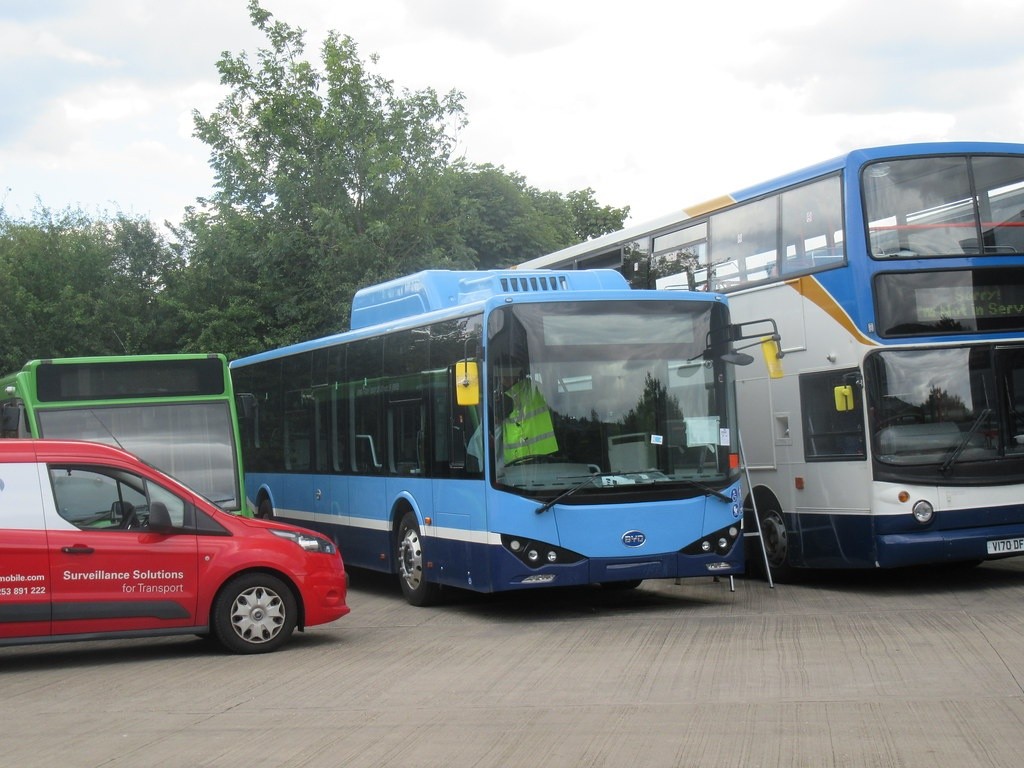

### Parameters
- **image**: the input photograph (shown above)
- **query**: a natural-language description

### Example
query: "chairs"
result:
[811,248,844,265]
[286,435,386,473]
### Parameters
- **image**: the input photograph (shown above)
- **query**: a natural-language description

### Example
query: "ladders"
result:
[674,418,776,592]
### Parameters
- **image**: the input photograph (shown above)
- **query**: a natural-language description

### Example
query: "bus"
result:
[513,140,1024,585]
[0,351,261,519]
[228,266,788,607]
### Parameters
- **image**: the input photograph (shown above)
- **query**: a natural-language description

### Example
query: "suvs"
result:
[0,436,353,654]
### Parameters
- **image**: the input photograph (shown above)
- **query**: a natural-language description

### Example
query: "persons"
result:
[495,356,559,464]
